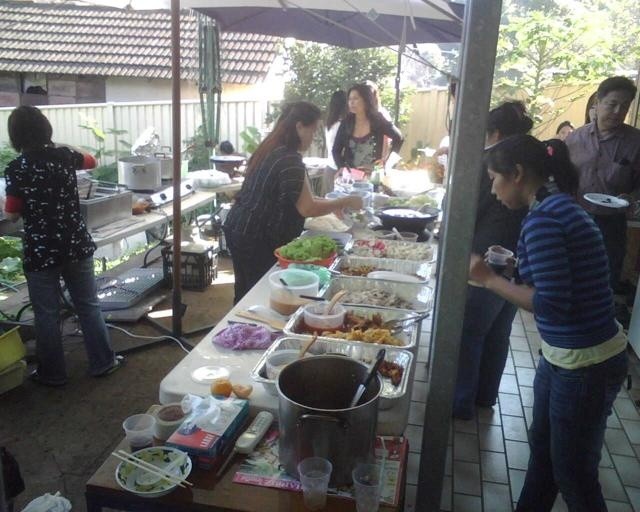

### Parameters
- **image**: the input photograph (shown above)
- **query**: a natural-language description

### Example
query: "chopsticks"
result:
[111,450,193,491]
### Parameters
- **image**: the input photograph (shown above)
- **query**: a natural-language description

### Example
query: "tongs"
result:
[384,312,431,335]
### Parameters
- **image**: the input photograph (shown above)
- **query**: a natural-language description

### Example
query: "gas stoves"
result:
[111,177,194,206]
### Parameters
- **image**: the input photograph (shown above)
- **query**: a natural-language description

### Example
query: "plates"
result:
[583,193,630,209]
[367,270,419,284]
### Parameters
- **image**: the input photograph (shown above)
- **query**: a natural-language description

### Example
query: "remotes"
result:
[234,411,274,455]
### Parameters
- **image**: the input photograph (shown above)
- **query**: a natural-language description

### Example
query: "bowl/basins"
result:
[303,300,347,331]
[265,348,313,380]
[156,402,187,438]
[115,447,193,499]
[267,268,320,315]
[371,203,442,231]
[273,246,338,270]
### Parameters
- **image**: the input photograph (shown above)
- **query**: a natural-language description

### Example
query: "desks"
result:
[199,161,324,198]
[0,189,216,323]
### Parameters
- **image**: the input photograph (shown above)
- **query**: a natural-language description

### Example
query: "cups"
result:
[486,245,513,276]
[122,413,156,453]
[352,464,384,512]
[296,456,334,511]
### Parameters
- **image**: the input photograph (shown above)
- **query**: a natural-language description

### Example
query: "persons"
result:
[333,86,404,176]
[556,121,574,141]
[323,91,347,193]
[1,104,127,387]
[468,135,629,512]
[453,102,533,420]
[585,92,599,124]
[220,141,243,156]
[563,76,639,283]
[360,81,392,164]
[222,103,364,306]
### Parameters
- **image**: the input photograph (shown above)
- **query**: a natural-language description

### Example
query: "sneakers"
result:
[101,354,125,377]
[30,369,64,384]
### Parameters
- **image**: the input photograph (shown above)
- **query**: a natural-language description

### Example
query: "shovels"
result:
[236,309,286,329]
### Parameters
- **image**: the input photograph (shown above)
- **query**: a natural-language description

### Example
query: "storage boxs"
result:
[1,362,26,394]
[0,325,27,374]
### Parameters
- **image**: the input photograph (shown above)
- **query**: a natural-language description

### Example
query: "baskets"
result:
[161,242,219,291]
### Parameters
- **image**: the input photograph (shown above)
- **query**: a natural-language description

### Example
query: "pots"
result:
[118,155,162,190]
[151,146,174,180]
[275,352,384,488]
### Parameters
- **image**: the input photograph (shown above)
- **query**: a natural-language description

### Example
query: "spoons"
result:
[136,451,188,485]
